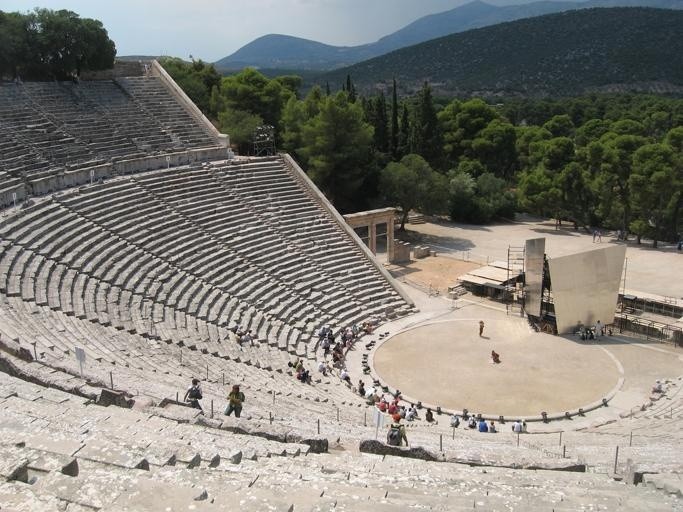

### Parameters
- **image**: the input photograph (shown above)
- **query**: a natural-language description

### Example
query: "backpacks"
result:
[230,392,245,402]
[386,424,404,446]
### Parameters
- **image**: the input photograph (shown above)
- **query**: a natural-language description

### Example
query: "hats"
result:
[393,414,401,420]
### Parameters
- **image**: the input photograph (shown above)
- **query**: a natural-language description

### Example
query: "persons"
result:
[235,324,254,346]
[224,385,243,417]
[387,414,408,446]
[293,322,527,433]
[184,379,204,415]
[589,320,604,341]
[651,379,662,394]
